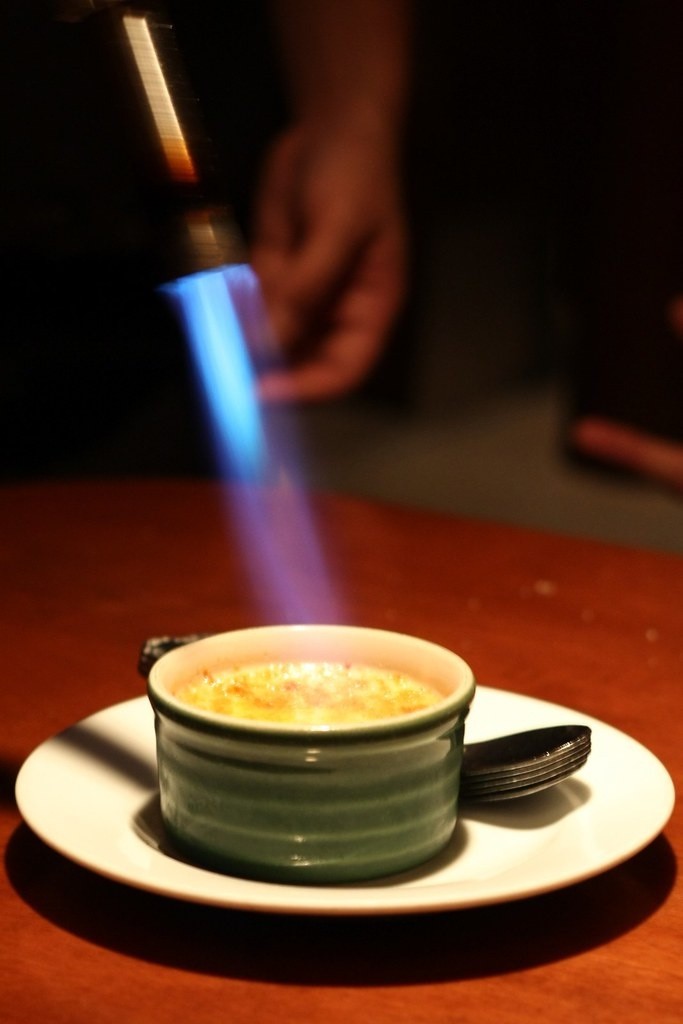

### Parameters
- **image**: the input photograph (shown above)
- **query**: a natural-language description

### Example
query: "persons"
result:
[257,0,414,402]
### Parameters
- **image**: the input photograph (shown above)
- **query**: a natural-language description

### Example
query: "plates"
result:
[16,686,675,918]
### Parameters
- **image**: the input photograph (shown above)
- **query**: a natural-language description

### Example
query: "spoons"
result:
[140,632,591,801]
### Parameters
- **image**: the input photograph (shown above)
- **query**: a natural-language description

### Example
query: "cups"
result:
[147,623,474,882]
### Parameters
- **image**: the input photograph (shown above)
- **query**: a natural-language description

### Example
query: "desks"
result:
[1,481,683,1024]
